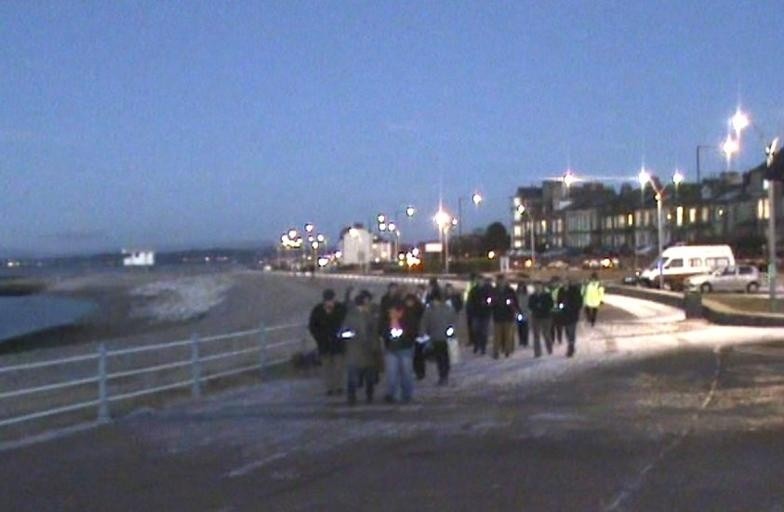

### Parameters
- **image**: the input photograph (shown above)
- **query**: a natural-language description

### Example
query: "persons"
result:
[309,273,606,405]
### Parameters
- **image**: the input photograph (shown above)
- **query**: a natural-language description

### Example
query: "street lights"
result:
[435,211,450,269]
[517,205,535,274]
[723,111,781,312]
[639,172,682,288]
[378,213,400,251]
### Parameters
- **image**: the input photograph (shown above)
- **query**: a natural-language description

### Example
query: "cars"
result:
[688,264,761,293]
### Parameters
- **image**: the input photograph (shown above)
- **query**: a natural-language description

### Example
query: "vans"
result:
[639,245,735,287]
